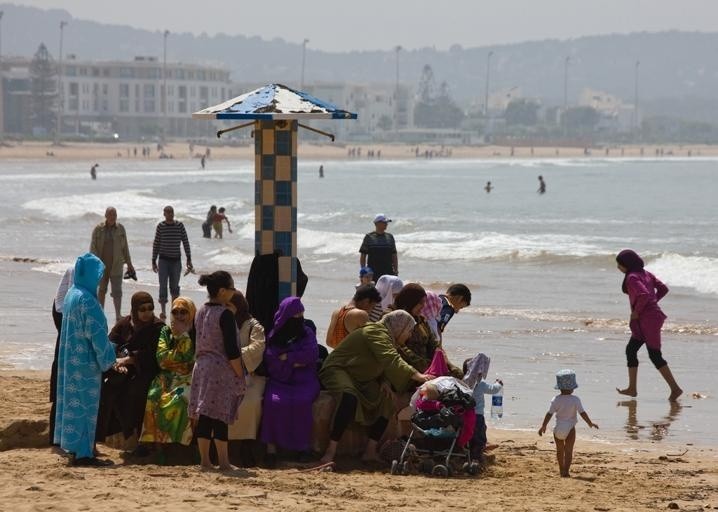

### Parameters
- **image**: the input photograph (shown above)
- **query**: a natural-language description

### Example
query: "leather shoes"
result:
[263,449,280,468]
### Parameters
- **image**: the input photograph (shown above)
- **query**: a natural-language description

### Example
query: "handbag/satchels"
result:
[108,344,136,383]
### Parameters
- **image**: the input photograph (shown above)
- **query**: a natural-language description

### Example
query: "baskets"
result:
[380,440,408,466]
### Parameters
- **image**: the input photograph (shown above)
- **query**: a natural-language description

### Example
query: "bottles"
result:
[490,381,504,420]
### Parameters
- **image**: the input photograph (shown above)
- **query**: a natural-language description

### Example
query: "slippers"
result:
[190,267,196,274]
[129,269,138,281]
[124,270,130,279]
[184,267,191,276]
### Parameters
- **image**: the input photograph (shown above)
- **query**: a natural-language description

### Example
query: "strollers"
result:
[390,348,489,479]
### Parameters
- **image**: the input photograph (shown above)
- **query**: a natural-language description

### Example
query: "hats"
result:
[359,267,374,276]
[373,213,392,224]
[554,369,578,390]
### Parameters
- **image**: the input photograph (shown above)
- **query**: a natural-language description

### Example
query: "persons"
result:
[613,249,685,403]
[89,206,136,322]
[359,213,398,283]
[96,267,504,473]
[52,254,127,469]
[111,141,213,171]
[49,265,76,443]
[536,176,545,193]
[201,205,223,238]
[318,165,323,176]
[538,369,598,478]
[212,207,232,239]
[345,144,694,162]
[150,206,193,317]
[616,399,682,443]
[90,163,98,179]
[484,181,493,192]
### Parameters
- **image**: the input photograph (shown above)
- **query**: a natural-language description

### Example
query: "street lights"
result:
[393,46,401,109]
[484,52,493,131]
[633,59,640,135]
[561,53,572,107]
[160,29,170,128]
[53,22,69,139]
[298,37,308,93]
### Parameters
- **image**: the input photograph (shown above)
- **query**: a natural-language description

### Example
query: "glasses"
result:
[139,306,154,312]
[173,309,186,314]
[226,287,237,291]
